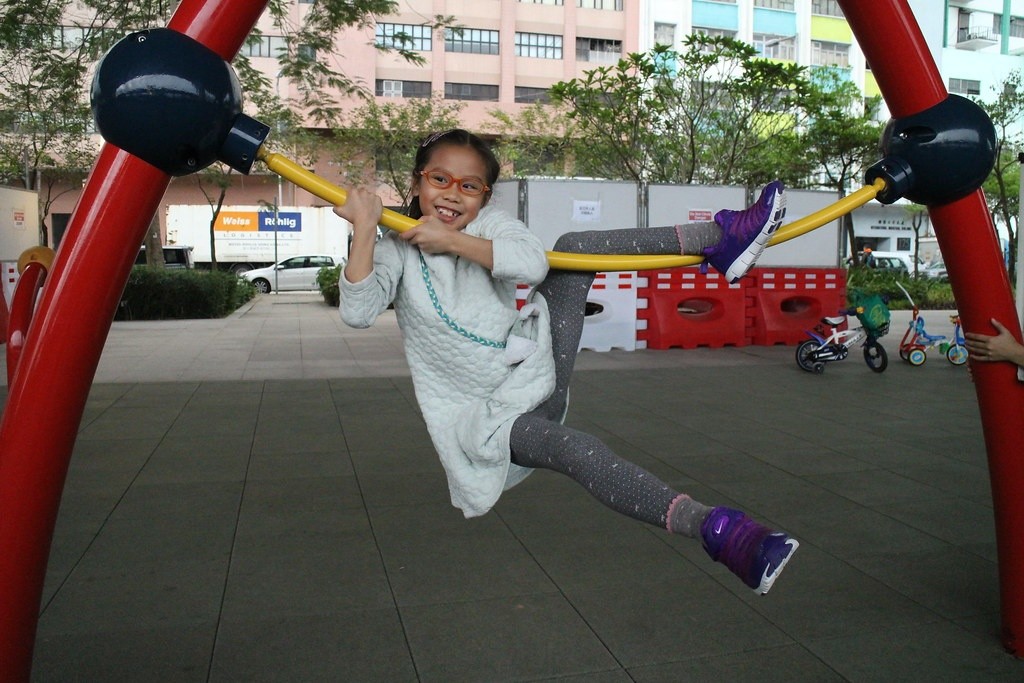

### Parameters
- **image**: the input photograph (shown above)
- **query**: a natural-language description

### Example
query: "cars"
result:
[911,260,948,281]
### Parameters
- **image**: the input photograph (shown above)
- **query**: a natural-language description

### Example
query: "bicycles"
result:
[795,297,889,375]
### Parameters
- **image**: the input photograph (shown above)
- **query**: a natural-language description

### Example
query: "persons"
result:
[965,318,1024,366]
[861,244,875,269]
[333,128,800,597]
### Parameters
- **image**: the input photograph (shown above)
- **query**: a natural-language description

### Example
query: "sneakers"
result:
[697,181,787,284]
[701,507,799,595]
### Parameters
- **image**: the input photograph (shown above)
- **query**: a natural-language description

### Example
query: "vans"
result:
[133,245,196,270]
[844,251,926,276]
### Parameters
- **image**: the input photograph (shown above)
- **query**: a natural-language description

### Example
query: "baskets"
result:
[864,321,891,340]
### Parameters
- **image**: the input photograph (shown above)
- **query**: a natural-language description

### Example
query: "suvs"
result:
[239,254,348,294]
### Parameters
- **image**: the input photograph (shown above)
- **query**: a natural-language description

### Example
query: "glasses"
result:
[417,169,491,195]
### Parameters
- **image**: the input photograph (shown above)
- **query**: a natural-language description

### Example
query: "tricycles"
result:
[892,276,969,367]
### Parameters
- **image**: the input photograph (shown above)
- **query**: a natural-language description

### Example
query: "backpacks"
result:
[854,295,890,333]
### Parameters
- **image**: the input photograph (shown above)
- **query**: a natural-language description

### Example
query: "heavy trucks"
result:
[166,204,384,278]
